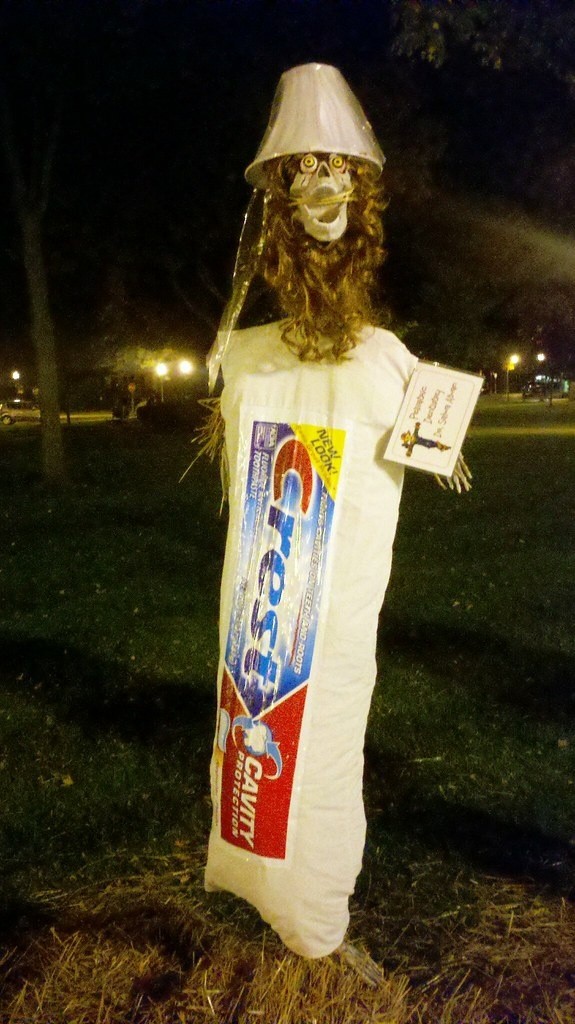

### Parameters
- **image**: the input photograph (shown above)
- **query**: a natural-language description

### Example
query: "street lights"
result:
[505,355,518,401]
[157,364,167,403]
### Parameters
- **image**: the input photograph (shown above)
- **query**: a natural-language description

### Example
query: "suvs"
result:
[0,401,40,425]
